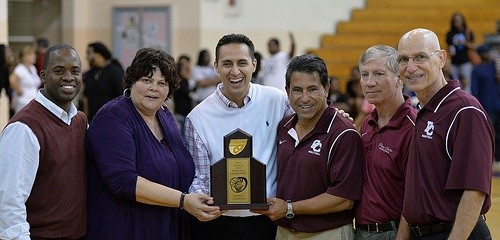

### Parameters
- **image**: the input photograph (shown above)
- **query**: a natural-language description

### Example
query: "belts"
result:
[358,223,393,233]
[411,225,451,237]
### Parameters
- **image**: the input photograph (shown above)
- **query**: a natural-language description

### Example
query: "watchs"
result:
[286,200,294,219]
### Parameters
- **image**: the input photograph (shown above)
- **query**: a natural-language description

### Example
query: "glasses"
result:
[399,50,439,64]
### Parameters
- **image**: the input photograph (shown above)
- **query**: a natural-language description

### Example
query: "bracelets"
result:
[180,193,185,210]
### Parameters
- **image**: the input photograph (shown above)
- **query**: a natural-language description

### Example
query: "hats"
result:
[477,45,492,54]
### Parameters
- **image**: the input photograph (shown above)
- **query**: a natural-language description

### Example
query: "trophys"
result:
[209,128,270,210]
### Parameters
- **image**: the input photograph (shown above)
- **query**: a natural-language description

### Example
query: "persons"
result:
[446,12,500,176]
[184,34,357,240]
[82,42,126,124]
[352,45,419,240]
[0,39,49,118]
[174,49,220,126]
[0,45,90,240]
[84,48,219,240]
[247,54,363,240]
[394,28,494,240]
[252,31,295,91]
[328,67,375,134]
